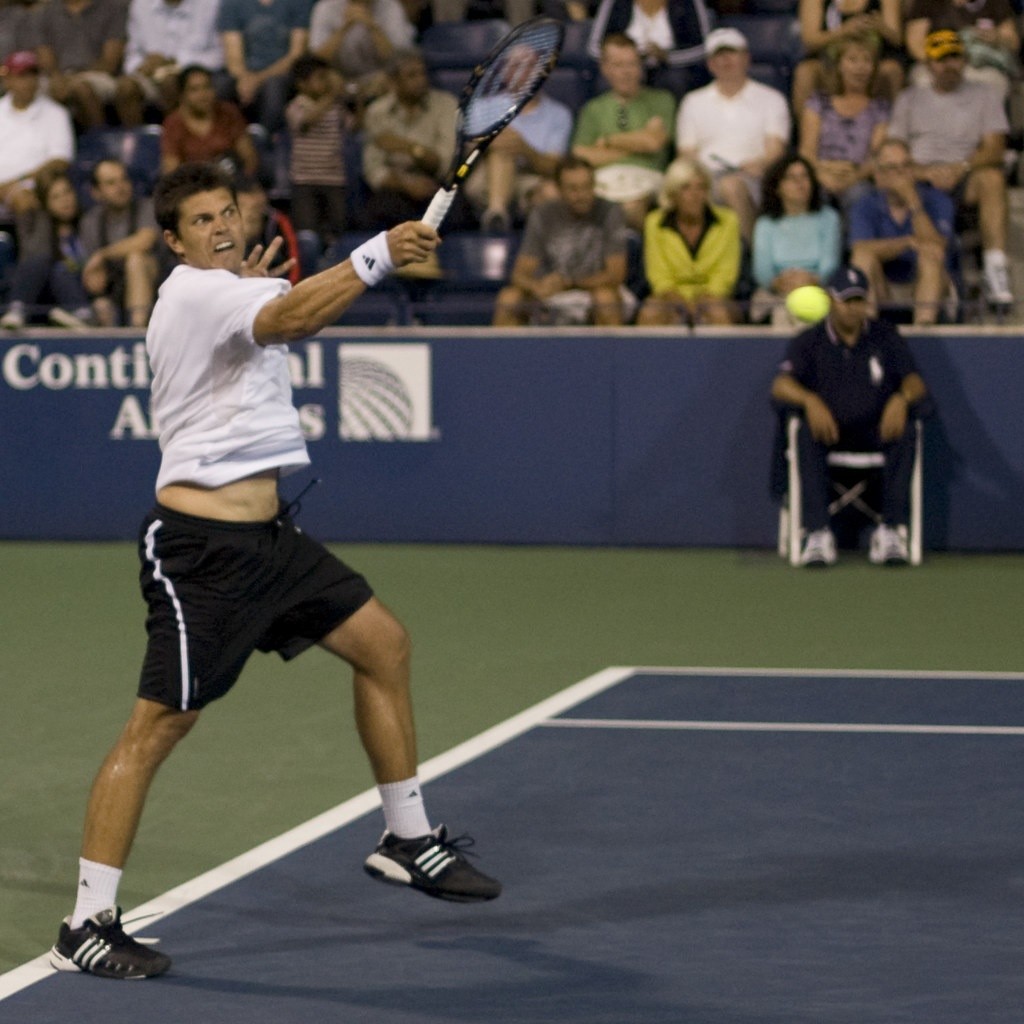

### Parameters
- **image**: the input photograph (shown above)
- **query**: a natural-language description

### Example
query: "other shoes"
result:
[800,527,836,567]
[4,308,26,327]
[982,264,1015,304]
[869,524,909,565]
[482,211,510,236]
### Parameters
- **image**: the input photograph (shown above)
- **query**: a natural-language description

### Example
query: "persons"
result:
[766,264,928,569]
[49,157,502,983]
[0,0,1023,332]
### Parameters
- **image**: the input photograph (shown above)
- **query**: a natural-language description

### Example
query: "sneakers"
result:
[364,824,501,904]
[49,906,172,980]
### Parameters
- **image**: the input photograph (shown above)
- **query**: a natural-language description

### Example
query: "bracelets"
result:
[898,390,912,407]
[350,230,396,287]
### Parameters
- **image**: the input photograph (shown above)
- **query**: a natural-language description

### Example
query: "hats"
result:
[6,51,40,77]
[924,30,966,60]
[830,268,869,296]
[706,28,747,54]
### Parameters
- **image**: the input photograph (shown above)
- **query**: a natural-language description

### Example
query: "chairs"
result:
[778,415,923,568]
[298,229,520,328]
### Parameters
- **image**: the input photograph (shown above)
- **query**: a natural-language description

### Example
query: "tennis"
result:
[785,285,831,323]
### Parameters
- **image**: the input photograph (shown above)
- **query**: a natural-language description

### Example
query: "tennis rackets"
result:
[418,14,568,231]
